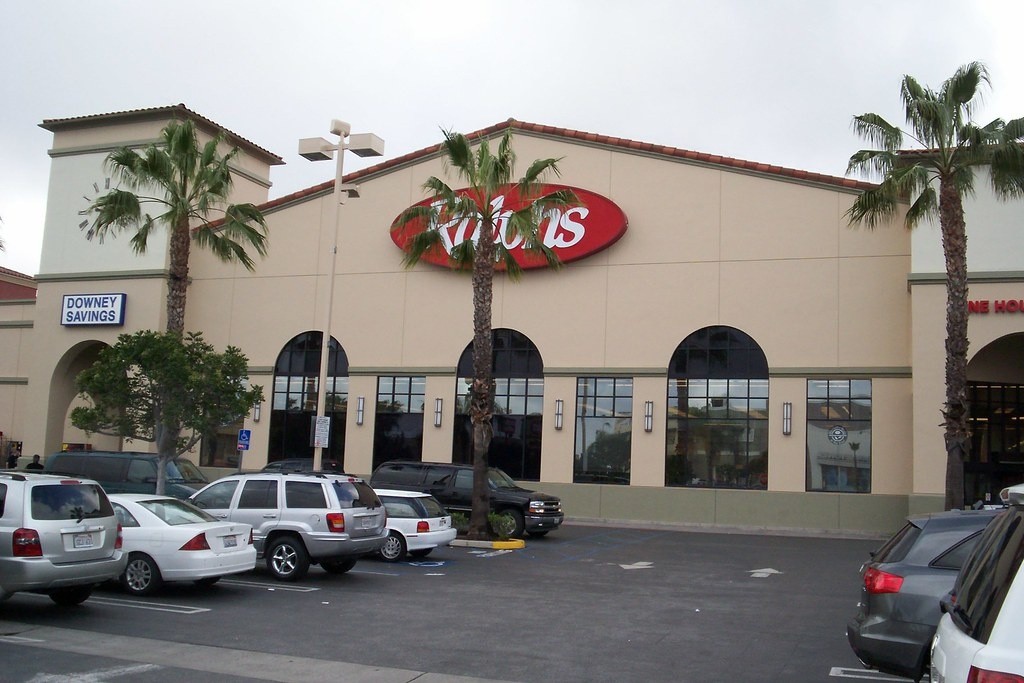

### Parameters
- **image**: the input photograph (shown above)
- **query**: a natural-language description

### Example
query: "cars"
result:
[345,487,458,563]
[77,493,258,597]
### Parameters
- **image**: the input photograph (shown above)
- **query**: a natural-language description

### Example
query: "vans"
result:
[41,451,232,509]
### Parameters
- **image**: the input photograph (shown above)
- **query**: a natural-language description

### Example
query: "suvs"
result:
[928,483,1024,683]
[0,468,130,608]
[180,468,391,582]
[369,460,565,539]
[845,507,1007,683]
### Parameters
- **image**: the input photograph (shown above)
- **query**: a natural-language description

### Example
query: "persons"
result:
[25,455,44,472]
[7,452,18,469]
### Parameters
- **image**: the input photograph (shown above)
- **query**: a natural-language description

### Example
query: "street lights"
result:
[297,118,385,470]
